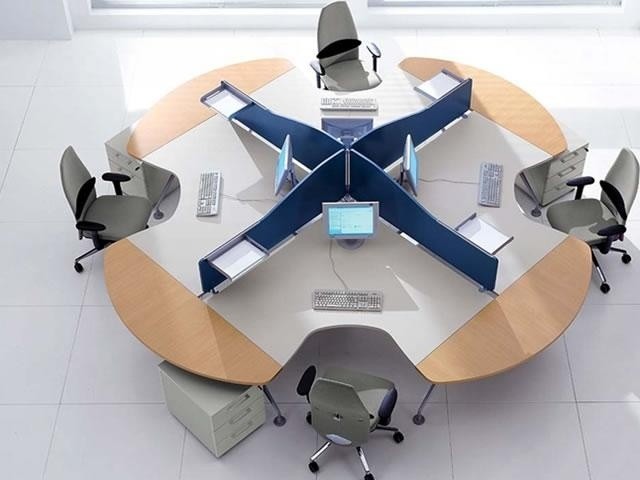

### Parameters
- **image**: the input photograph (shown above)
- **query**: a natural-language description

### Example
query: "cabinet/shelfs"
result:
[522,132,589,209]
[105,121,179,207]
[155,350,269,464]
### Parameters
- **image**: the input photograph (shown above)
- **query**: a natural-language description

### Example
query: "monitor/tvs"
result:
[399,133,419,197]
[274,134,299,198]
[321,117,373,145]
[322,201,380,252]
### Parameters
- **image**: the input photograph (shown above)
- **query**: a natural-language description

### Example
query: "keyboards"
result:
[312,288,383,312]
[320,97,378,111]
[196,171,220,216]
[478,161,503,207]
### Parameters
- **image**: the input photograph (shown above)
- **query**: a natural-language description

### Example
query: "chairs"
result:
[58,143,155,275]
[309,0,387,96]
[290,364,408,479]
[546,145,640,293]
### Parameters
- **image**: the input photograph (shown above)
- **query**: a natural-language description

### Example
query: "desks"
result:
[99,51,597,426]
